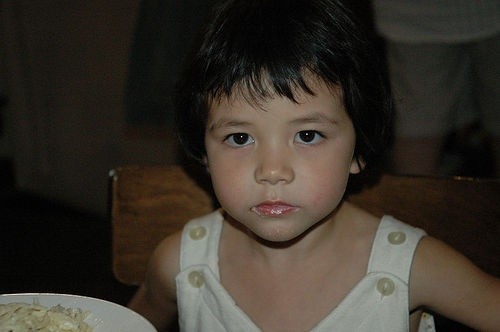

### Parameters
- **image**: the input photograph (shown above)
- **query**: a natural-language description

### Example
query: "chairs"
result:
[109,165,497,290]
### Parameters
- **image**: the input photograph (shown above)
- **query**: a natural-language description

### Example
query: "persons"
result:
[127,0,500,332]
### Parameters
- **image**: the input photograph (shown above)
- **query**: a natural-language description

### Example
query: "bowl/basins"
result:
[0,294,157,332]
